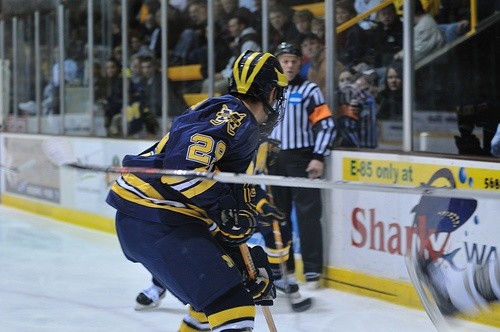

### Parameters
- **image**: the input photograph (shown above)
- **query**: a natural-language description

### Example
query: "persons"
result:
[136,135,288,306]
[106,49,289,332]
[418,243,500,315]
[270,41,338,281]
[0,0,500,158]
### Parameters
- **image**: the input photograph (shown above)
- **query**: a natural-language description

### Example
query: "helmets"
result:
[227,49,288,101]
[274,40,302,58]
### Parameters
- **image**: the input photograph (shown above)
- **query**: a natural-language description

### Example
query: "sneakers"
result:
[305,272,321,289]
[273,278,301,299]
[134,284,166,311]
[404,253,460,332]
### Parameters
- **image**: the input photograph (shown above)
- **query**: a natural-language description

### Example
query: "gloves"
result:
[253,189,288,228]
[243,245,277,306]
[214,201,259,246]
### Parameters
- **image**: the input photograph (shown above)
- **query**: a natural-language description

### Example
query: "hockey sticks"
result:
[263,168,312,312]
[239,240,277,332]
[63,160,500,202]
